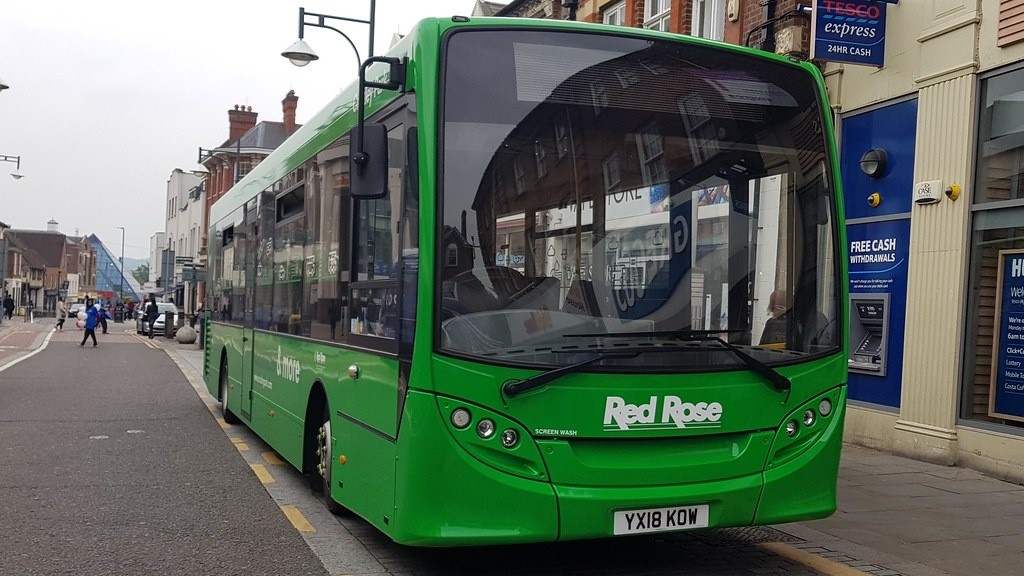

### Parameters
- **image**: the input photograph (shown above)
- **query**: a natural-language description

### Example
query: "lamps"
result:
[867,192,880,208]
[944,184,960,201]
[859,148,887,178]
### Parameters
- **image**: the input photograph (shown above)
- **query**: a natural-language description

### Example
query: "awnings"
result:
[157,287,183,295]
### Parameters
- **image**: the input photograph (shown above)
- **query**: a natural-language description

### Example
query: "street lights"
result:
[189,137,241,187]
[115,226,125,302]
[278,0,379,72]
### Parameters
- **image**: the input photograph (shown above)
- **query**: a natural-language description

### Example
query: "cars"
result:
[136,302,180,337]
[67,304,85,318]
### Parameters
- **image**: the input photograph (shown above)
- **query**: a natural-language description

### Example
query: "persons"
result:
[222,300,232,323]
[365,295,386,333]
[3,294,15,319]
[760,291,788,344]
[146,299,158,339]
[116,298,141,321]
[54,297,66,331]
[328,299,338,339]
[169,298,174,303]
[76,299,97,348]
[256,300,301,335]
[95,307,110,334]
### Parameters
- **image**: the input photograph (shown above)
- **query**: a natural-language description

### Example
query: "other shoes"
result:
[91,345,98,348]
[77,343,84,347]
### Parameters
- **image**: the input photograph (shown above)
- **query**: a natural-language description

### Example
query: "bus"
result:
[202,16,856,549]
